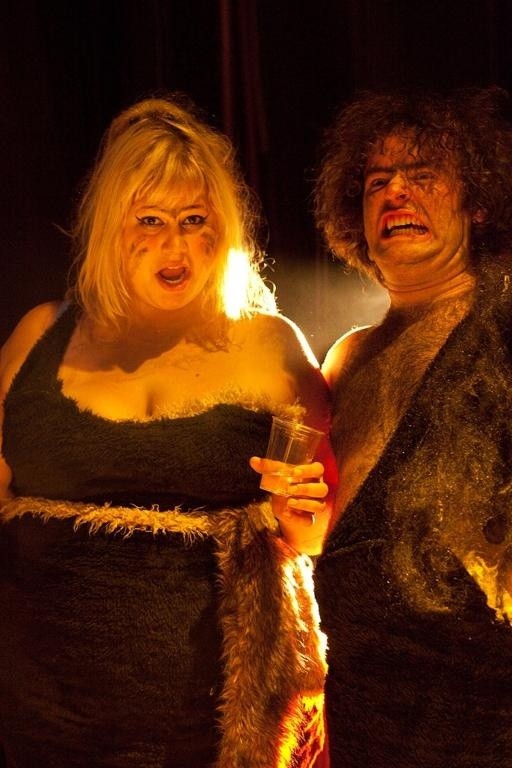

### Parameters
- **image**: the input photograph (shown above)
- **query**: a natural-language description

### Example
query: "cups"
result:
[258,414,326,501]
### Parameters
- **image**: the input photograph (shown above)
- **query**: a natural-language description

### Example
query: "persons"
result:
[0,96,343,767]
[309,83,510,765]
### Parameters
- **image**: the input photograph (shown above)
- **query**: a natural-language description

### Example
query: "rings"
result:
[311,514,315,528]
